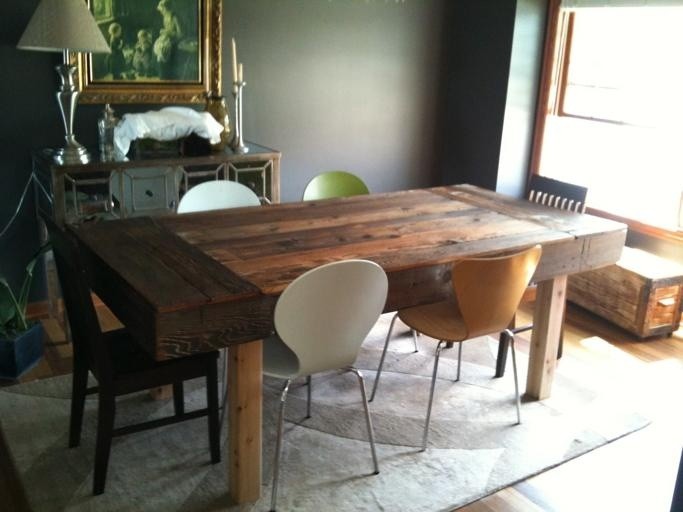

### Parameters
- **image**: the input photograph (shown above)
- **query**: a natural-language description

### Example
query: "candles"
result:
[231,36,237,93]
[238,63,243,82]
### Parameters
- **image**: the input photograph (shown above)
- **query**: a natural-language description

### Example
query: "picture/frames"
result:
[68,0,222,104]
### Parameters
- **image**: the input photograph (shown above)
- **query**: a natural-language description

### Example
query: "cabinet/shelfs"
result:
[30,137,282,343]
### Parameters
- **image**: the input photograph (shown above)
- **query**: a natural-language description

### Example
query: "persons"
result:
[104,22,128,80]
[129,28,153,75]
[152,1,186,78]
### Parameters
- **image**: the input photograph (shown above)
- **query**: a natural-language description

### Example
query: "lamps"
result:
[16,0,112,167]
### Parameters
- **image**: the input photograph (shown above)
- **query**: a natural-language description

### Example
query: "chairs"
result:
[255,256,390,512]
[301,173,373,199]
[366,243,549,448]
[40,208,224,497]
[440,171,587,381]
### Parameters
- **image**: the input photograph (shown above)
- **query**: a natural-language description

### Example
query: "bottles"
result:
[98,103,119,162]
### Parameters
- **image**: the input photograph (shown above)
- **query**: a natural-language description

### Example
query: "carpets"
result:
[0,309,652,512]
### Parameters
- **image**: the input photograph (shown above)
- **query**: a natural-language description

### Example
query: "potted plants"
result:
[0,238,53,381]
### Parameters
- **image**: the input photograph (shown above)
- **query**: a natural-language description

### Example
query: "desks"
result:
[79,184,628,503]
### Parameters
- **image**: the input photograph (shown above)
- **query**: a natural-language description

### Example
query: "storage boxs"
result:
[565,246,683,340]
